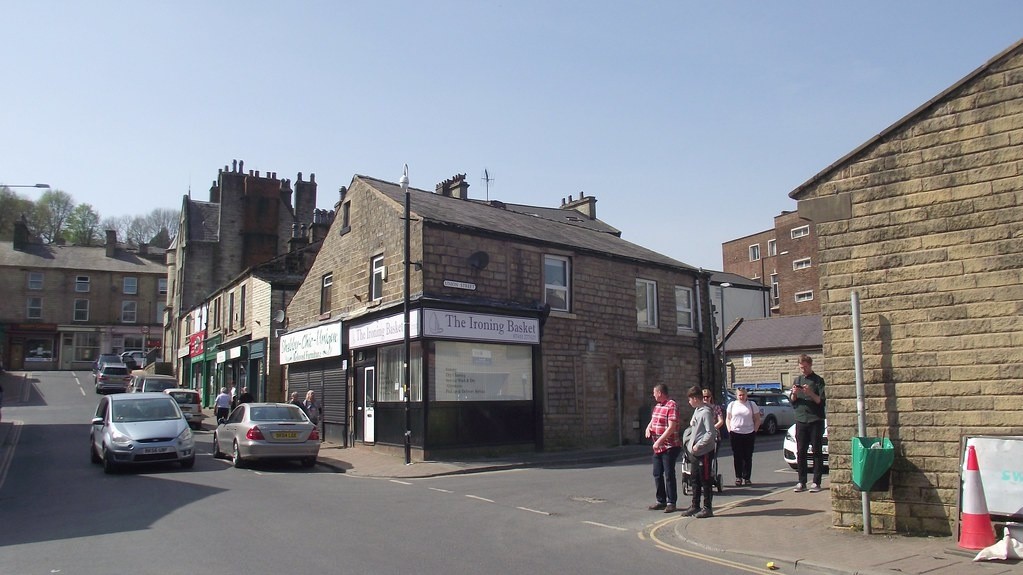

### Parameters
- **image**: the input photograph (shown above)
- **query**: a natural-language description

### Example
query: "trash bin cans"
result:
[853,437,894,491]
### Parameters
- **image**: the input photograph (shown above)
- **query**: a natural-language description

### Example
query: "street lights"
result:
[720,283,731,393]
[399,163,412,466]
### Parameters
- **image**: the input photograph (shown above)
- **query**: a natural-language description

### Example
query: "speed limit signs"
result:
[141,326,150,333]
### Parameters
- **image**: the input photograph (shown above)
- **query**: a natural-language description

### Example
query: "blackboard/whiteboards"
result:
[956,434,1023,518]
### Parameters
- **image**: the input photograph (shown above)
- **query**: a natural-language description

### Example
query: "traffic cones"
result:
[943,445,997,558]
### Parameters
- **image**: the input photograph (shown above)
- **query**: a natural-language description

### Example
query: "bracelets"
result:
[792,393,796,395]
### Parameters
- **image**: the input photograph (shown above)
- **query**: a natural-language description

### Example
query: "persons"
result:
[117,404,135,418]
[726,387,761,485]
[213,381,253,426]
[681,386,724,518]
[789,355,825,492]
[645,384,682,513]
[285,390,320,425]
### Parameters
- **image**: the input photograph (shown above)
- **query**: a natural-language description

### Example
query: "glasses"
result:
[703,395,710,398]
[738,387,745,389]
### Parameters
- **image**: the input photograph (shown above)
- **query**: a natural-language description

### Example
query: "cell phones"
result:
[795,384,803,388]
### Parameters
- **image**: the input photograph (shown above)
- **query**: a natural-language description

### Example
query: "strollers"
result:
[681,426,723,497]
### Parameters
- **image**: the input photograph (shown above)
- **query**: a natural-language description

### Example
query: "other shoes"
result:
[680,505,701,516]
[735,478,742,485]
[664,505,676,513]
[695,506,713,518]
[794,482,806,491]
[809,483,822,491]
[745,479,751,485]
[648,500,667,510]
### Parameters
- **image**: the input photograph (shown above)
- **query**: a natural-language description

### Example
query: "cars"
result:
[93,353,123,373]
[89,392,195,474]
[747,393,795,435]
[125,375,180,394]
[121,351,148,368]
[95,362,131,394]
[783,419,829,470]
[164,388,202,430]
[212,402,321,469]
